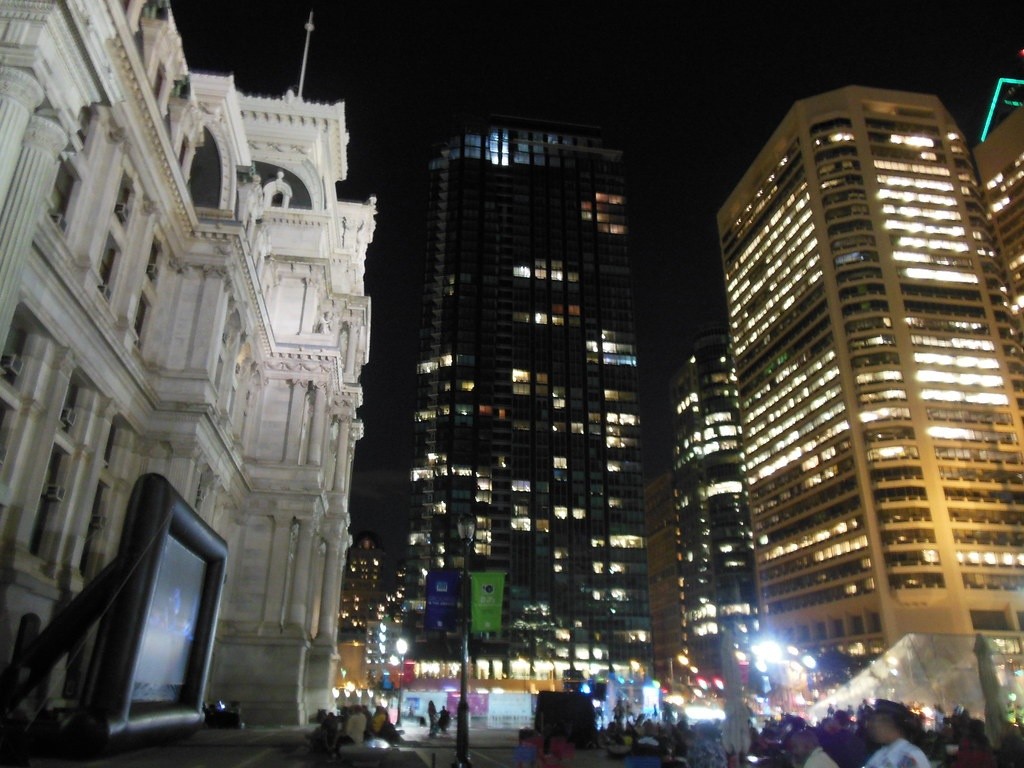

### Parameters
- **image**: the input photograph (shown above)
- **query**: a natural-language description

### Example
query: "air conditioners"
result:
[46,485,66,502]
[91,515,106,528]
[147,264,159,279]
[0,354,24,376]
[98,285,112,301]
[61,407,77,426]
[51,213,67,232]
[114,203,128,221]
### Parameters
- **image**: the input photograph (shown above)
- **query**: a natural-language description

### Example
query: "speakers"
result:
[595,683,607,701]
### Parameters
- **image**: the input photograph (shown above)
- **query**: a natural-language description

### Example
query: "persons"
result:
[594,692,1022,768]
[334,705,366,760]
[543,730,575,767]
[513,727,540,768]
[863,711,927,767]
[783,728,841,767]
[320,711,333,748]
[427,699,438,736]
[439,706,453,731]
[215,700,227,711]
[368,705,388,734]
